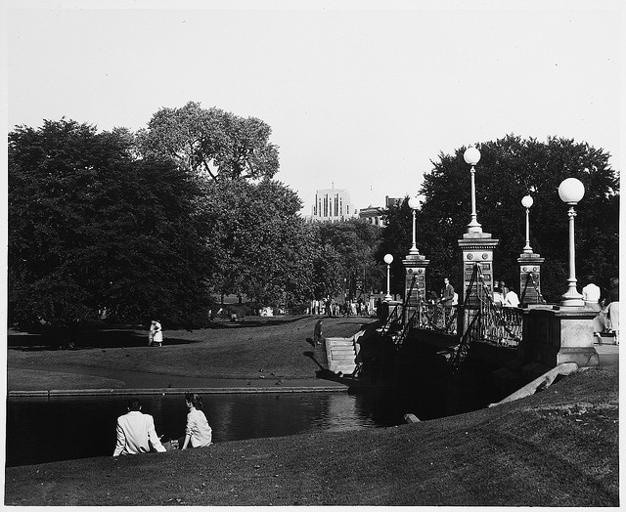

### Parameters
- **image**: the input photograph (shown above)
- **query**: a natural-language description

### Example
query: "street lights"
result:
[520,193,535,254]
[557,177,585,306]
[462,145,484,234]
[408,194,423,255]
[383,254,393,301]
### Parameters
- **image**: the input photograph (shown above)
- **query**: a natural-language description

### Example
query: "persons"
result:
[493,280,521,307]
[113,393,213,457]
[313,319,323,345]
[425,276,459,330]
[582,275,619,346]
[148,319,163,347]
